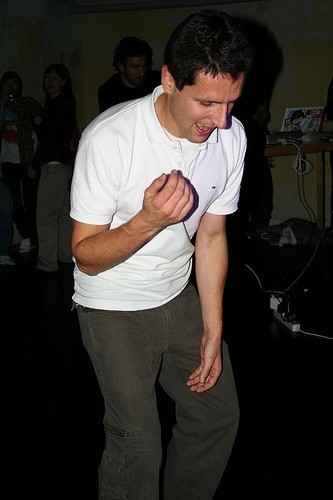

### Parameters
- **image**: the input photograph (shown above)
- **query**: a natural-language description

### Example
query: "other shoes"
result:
[60,259,76,271]
[19,238,31,253]
[34,257,57,274]
[0,255,16,266]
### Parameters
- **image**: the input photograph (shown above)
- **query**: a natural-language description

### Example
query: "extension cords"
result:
[274,310,300,331]
